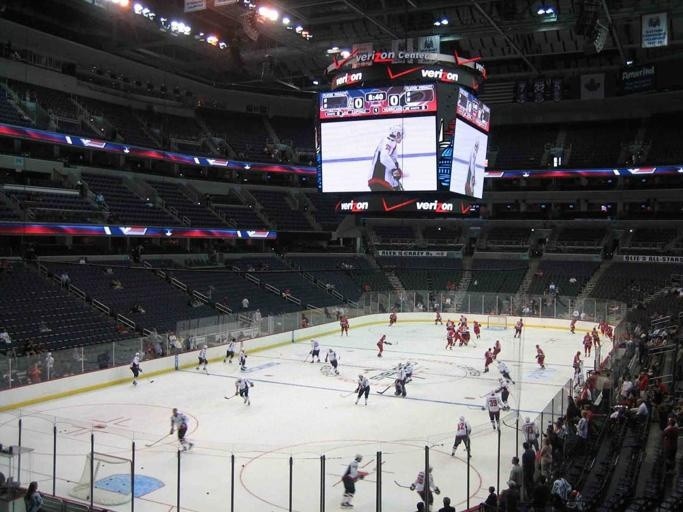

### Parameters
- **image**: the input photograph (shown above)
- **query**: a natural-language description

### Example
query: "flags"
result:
[593,25,608,54]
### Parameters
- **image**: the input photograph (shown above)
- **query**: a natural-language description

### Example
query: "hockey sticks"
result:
[502,419,522,431]
[394,480,439,493]
[376,380,396,394]
[340,388,359,398]
[142,371,154,383]
[145,433,170,447]
[224,386,251,399]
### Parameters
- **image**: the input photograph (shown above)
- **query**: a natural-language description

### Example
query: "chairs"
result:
[468,448,681,510]
[173,77,359,352]
[543,319,681,448]
[2,478,109,511]
[359,77,682,319]
[1,76,173,392]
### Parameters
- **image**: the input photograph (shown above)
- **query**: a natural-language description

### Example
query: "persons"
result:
[0,41,683,512]
[648,19,659,30]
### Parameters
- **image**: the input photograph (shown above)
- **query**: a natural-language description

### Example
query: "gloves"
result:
[392,168,402,180]
[473,177,475,185]
[410,483,416,491]
[434,489,440,494]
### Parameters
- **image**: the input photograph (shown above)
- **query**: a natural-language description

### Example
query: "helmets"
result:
[475,141,480,151]
[388,121,406,142]
[459,417,464,421]
[356,454,363,462]
